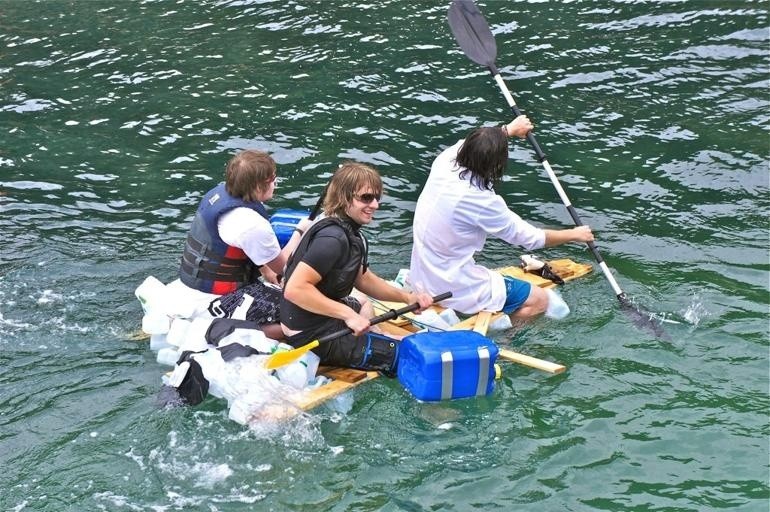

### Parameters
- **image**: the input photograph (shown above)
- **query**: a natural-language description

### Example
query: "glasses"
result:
[351,192,381,206]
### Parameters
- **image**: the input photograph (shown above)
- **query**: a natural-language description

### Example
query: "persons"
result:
[170,150,318,342]
[410,114,596,330]
[281,162,437,378]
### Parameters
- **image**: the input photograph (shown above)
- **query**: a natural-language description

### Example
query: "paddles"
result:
[447,0,672,345]
[265,291,452,369]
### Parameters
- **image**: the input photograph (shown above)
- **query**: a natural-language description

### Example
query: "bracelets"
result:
[292,226,306,237]
[503,123,511,136]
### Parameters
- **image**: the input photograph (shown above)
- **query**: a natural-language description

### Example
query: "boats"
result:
[245,256,594,427]
